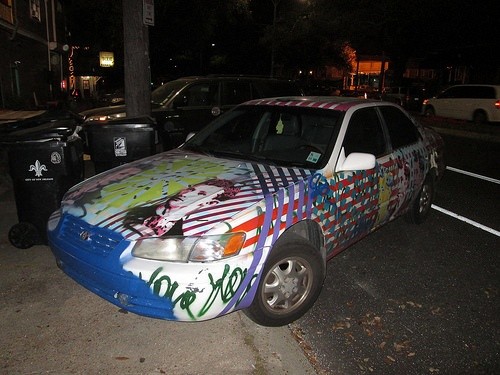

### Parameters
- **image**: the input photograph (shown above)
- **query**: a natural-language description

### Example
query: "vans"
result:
[420,83,500,125]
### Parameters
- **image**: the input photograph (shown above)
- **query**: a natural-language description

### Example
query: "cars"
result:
[44,94,448,329]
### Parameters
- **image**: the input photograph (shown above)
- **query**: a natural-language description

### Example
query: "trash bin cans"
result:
[2,119,85,248]
[86,116,157,175]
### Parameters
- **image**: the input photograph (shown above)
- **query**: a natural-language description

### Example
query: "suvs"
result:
[76,74,310,160]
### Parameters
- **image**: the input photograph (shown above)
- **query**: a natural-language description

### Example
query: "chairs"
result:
[255,114,340,154]
